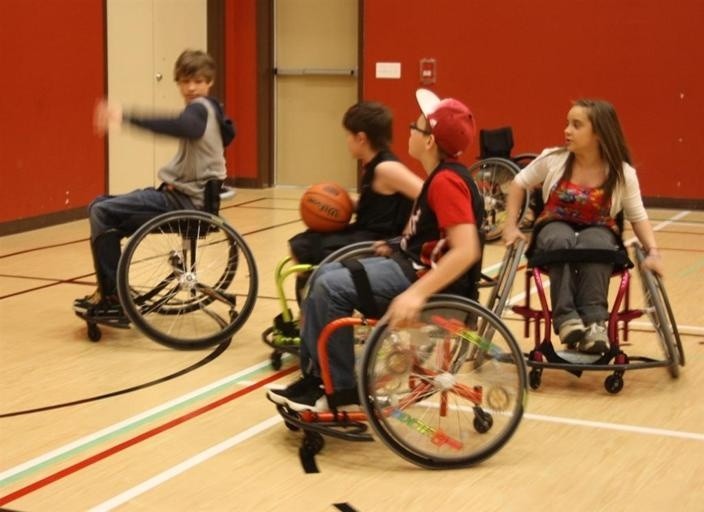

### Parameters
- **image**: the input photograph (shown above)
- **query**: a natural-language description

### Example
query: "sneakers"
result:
[558,318,611,353]
[73,289,122,314]
[267,376,360,413]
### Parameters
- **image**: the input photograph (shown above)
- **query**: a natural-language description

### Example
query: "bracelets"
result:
[646,246,658,252]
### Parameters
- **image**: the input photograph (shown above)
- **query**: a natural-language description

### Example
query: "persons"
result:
[501,98,664,352]
[73,50,234,315]
[265,101,424,344]
[267,86,485,414]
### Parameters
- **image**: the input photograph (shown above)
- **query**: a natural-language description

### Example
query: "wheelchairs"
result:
[70,177,258,350]
[465,125,554,243]
[273,230,531,472]
[474,236,687,396]
[260,241,437,376]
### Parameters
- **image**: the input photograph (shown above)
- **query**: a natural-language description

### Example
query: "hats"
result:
[416,88,475,159]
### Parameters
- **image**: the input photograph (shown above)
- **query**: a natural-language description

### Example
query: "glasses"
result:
[410,121,429,135]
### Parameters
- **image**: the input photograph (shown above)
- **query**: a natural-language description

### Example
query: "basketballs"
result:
[301,181,354,232]
